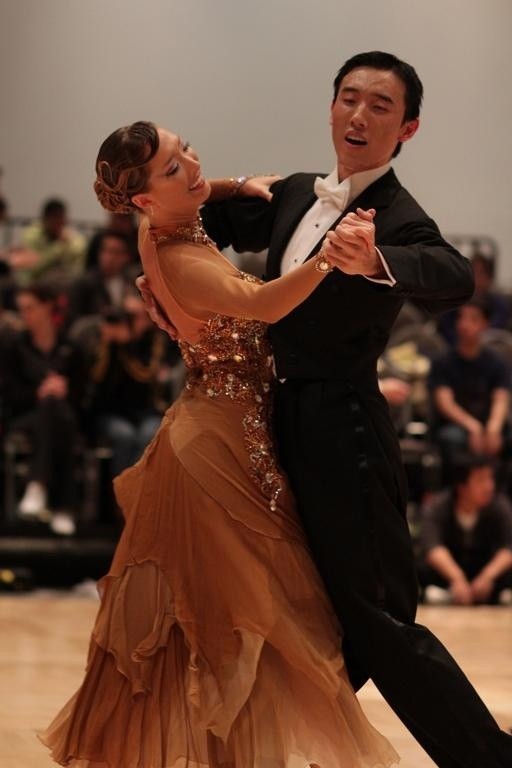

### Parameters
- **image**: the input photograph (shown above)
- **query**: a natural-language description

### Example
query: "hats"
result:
[377,342,431,375]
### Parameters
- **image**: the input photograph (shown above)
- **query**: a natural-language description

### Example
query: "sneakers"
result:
[424,585,454,606]
[17,481,77,534]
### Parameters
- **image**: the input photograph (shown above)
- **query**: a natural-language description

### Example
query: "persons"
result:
[201,54,511,768]
[0,197,511,606]
[38,121,402,768]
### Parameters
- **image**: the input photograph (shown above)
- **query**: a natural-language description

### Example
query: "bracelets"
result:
[227,174,257,193]
[314,253,333,273]
